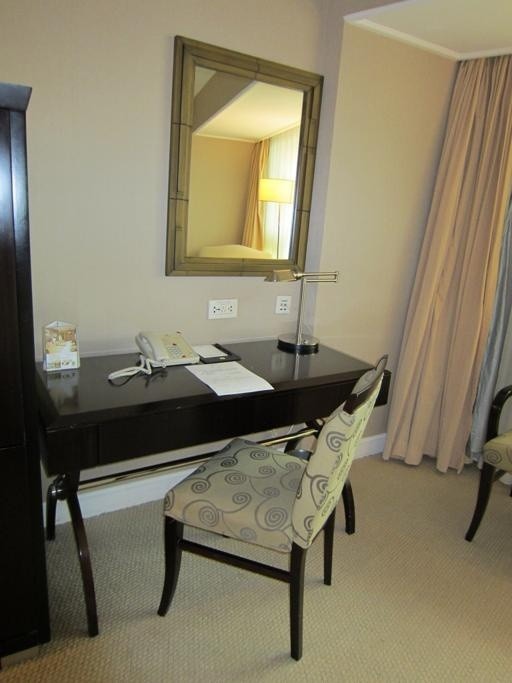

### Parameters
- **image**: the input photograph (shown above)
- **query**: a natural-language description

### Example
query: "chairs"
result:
[465,386,512,541]
[158,353,390,660]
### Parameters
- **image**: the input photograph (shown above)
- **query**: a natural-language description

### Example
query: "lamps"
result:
[263,267,339,354]
[257,178,292,259]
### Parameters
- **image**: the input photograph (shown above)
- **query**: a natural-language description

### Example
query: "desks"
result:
[33,336,392,637]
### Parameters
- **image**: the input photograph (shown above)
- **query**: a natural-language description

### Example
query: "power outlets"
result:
[208,299,238,320]
[275,296,292,314]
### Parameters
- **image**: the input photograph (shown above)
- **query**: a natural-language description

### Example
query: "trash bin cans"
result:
[286,449,313,465]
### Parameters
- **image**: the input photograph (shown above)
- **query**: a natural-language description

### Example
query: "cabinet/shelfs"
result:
[0,84,50,657]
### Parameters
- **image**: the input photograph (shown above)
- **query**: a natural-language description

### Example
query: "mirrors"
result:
[165,35,324,279]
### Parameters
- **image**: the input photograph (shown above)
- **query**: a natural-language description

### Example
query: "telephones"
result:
[136,330,198,366]
